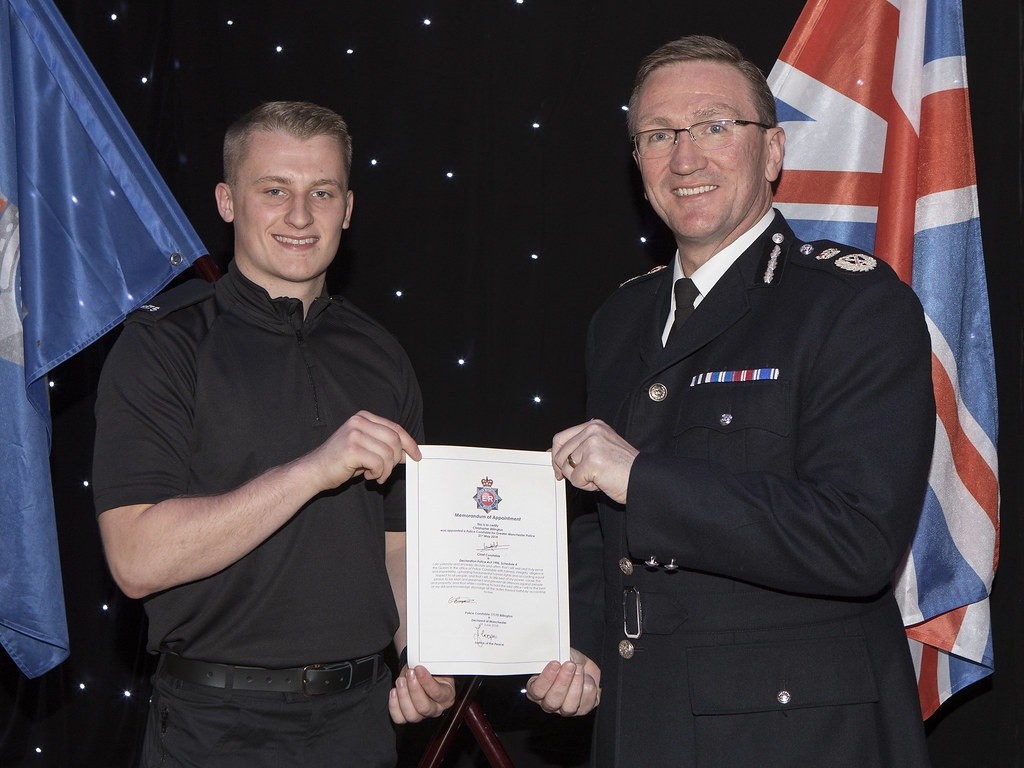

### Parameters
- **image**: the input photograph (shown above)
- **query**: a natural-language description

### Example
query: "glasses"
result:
[630,119,773,159]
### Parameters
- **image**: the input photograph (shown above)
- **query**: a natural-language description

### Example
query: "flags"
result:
[0,0,209,679]
[764,1,1001,719]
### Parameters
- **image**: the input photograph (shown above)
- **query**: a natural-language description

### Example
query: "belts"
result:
[166,651,381,698]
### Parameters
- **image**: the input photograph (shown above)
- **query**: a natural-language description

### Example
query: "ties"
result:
[665,278,700,348]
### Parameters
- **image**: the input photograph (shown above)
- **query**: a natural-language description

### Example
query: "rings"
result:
[568,452,576,468]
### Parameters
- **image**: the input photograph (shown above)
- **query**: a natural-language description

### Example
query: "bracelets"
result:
[393,644,408,669]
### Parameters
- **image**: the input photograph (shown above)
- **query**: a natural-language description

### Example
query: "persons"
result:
[93,36,937,768]
